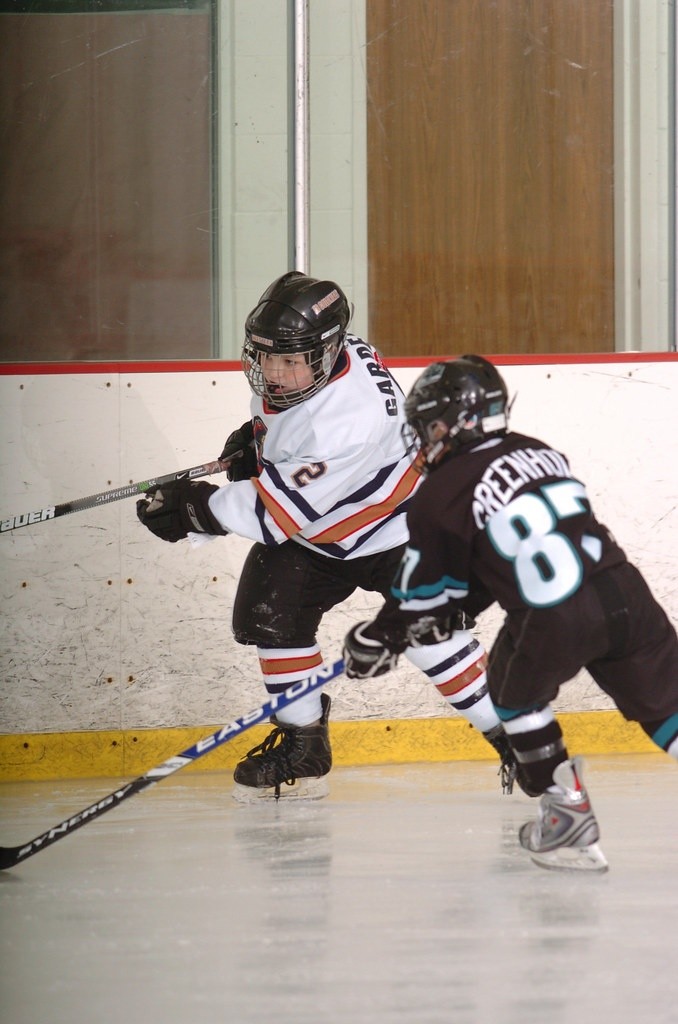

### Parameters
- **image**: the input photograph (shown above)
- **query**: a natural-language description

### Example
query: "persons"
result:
[341,353,678,853]
[136,271,548,797]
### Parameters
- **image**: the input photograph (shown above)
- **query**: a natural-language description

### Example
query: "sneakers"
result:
[482,722,543,798]
[231,693,331,804]
[519,755,608,870]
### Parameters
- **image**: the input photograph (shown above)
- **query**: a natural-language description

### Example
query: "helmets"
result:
[241,270,355,408]
[400,353,518,479]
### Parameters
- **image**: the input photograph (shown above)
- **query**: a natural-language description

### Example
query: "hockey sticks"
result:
[0,614,439,872]
[0,447,248,536]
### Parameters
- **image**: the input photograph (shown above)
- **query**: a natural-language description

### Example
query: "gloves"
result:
[403,607,477,649]
[136,479,228,542]
[217,420,261,483]
[342,621,398,680]
